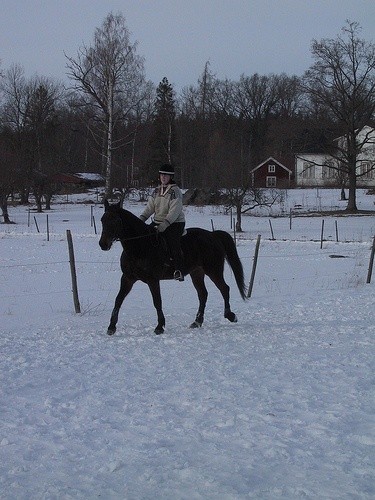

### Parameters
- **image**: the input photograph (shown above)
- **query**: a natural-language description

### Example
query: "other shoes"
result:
[174,270,184,282]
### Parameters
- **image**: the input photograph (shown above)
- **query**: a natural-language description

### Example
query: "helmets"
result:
[159,163,175,175]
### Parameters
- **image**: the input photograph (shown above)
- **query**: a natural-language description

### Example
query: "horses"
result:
[99,193,249,336]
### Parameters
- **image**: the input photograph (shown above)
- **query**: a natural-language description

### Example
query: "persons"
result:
[139,164,186,282]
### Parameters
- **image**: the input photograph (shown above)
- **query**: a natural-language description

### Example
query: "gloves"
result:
[158,220,170,232]
[140,216,146,222]
